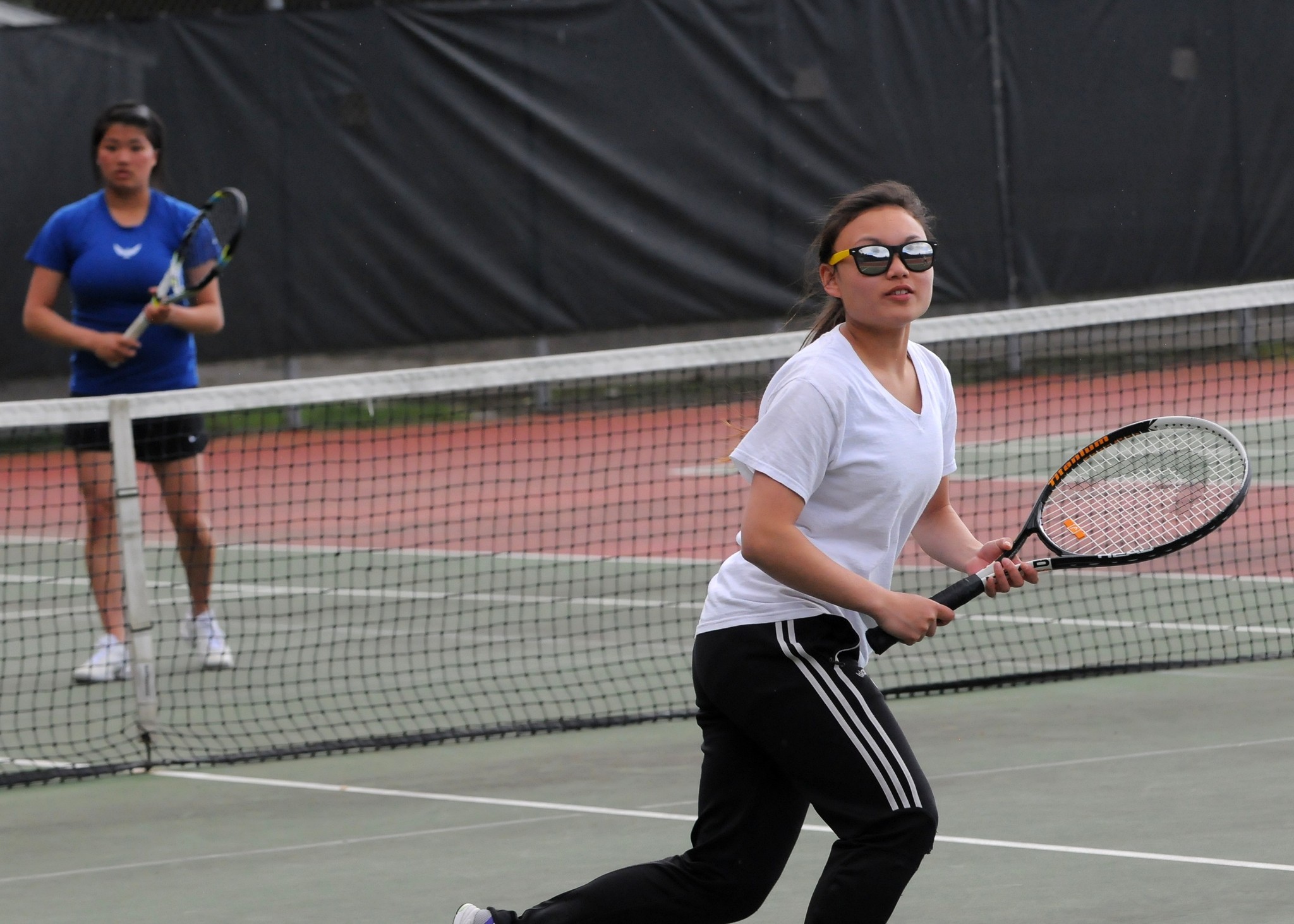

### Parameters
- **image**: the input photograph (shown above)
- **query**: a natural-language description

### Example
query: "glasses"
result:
[830,240,940,277]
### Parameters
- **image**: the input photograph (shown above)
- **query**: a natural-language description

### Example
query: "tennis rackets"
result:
[121,186,249,341]
[864,415,1251,655]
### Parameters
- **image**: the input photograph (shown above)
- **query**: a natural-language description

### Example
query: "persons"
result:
[23,103,232,682]
[454,180,1038,924]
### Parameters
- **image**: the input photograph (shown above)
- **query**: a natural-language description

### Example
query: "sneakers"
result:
[178,611,234,667]
[74,634,133,682]
